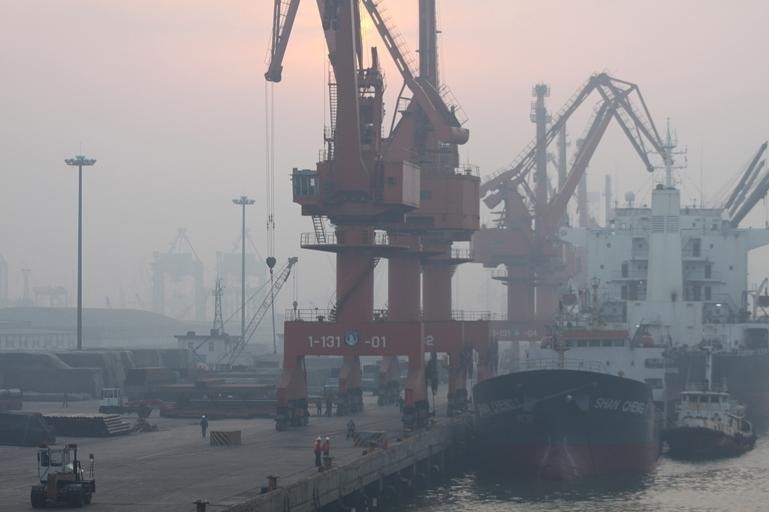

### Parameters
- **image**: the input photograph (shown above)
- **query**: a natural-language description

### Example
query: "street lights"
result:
[65,154,95,349]
[231,196,255,354]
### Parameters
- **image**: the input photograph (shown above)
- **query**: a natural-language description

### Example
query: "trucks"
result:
[99,388,152,416]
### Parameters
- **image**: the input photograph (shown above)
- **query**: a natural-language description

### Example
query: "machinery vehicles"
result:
[0,388,23,411]
[29,442,97,508]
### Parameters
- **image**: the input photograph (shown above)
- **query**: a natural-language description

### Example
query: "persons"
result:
[320,437,331,464]
[315,397,322,416]
[323,397,334,416]
[199,414,210,439]
[313,437,322,467]
[345,418,355,439]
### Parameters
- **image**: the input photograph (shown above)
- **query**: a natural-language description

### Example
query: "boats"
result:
[465,370,662,485]
[665,345,761,458]
